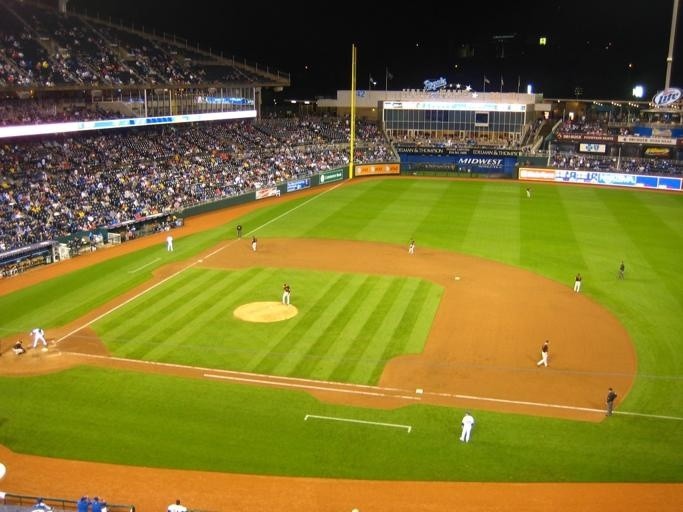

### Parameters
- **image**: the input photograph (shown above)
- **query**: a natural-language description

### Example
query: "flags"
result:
[484,76,489,83]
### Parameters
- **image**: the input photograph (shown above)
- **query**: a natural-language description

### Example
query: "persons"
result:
[603,387,617,416]
[1,0,260,279]
[76,495,92,512]
[281,281,290,306]
[615,260,625,280]
[407,237,415,254]
[166,498,187,512]
[33,497,54,512]
[571,272,581,292]
[458,411,476,442]
[11,340,25,355]
[535,339,550,367]
[261,108,397,198]
[524,186,532,198]
[91,496,106,512]
[28,328,48,349]
[398,104,681,178]
